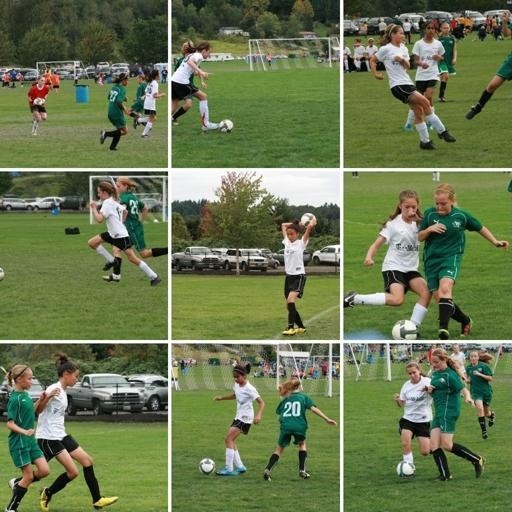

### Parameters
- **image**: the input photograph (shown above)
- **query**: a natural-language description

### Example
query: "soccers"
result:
[219,119,233,133]
[392,319,417,339]
[199,459,215,476]
[1,266,4,281]
[34,98,42,105]
[397,461,416,477]
[302,213,316,227]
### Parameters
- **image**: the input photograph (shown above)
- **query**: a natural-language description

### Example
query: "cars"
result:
[0,193,163,215]
[127,375,168,411]
[344,344,512,353]
[343,9,512,37]
[1,60,155,82]
[1,376,47,417]
[172,244,340,271]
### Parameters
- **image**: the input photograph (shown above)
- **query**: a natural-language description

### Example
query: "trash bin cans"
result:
[75,84,89,103]
[52,206,61,216]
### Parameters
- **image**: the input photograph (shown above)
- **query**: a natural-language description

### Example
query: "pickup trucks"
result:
[64,373,145,416]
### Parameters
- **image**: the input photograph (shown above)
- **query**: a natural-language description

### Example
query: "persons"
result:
[425,348,487,482]
[1,9,511,396]
[36,350,120,511]
[462,351,496,439]
[393,362,434,470]
[265,376,338,480]
[214,361,265,478]
[4,363,50,512]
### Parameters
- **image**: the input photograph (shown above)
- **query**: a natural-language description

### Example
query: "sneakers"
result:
[93,496,118,511]
[283,329,296,336]
[202,123,218,130]
[101,260,161,286]
[438,131,457,143]
[405,124,415,133]
[100,130,105,144]
[172,118,179,125]
[435,411,495,483]
[295,328,307,334]
[133,117,137,129]
[420,141,437,151]
[214,466,311,483]
[344,291,474,340]
[6,478,52,512]
[427,122,433,131]
[467,108,482,121]
